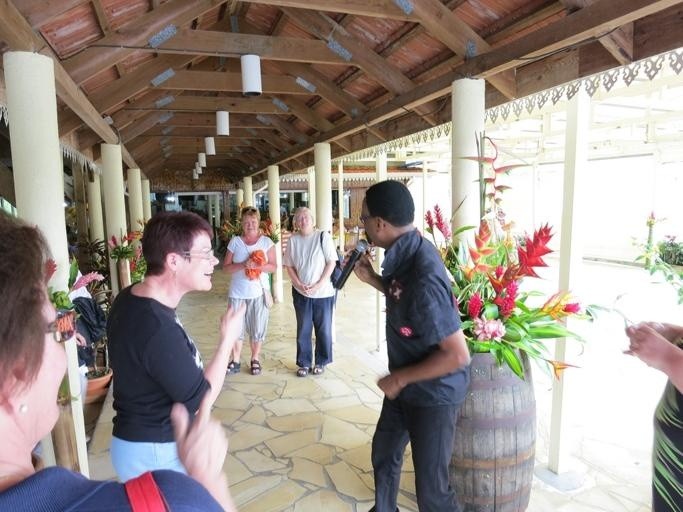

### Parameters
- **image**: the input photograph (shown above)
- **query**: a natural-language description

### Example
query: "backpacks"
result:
[331,247,344,286]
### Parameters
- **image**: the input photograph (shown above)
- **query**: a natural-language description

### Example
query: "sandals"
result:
[226,362,241,374]
[251,361,262,375]
[297,366,310,378]
[313,363,325,374]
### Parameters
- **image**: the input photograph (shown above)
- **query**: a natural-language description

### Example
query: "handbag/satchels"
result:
[124,471,173,512]
[241,234,274,309]
[319,231,344,289]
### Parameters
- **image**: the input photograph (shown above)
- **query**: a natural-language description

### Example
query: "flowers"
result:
[260,218,283,244]
[108,227,144,261]
[42,253,106,320]
[425,132,597,380]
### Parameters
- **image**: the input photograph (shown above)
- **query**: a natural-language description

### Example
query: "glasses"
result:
[359,214,378,224]
[42,312,76,345]
[177,249,215,262]
[242,208,259,221]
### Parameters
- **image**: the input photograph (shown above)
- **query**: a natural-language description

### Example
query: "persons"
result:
[222,206,277,374]
[67,264,97,366]
[0,208,235,512]
[341,180,472,512]
[107,209,248,483]
[284,207,337,377]
[621,318,683,512]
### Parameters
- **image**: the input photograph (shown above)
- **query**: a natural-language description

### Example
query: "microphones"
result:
[336,239,367,289]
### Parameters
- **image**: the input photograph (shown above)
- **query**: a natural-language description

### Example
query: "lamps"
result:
[193,169,199,180]
[198,153,206,167]
[241,54,262,97]
[195,162,202,174]
[204,137,216,156]
[216,111,230,136]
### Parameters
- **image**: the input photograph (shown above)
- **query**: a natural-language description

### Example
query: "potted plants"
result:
[69,232,82,246]
[634,234,683,304]
[85,275,113,405]
[75,232,107,270]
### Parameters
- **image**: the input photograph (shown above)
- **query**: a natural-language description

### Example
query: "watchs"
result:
[396,372,408,388]
